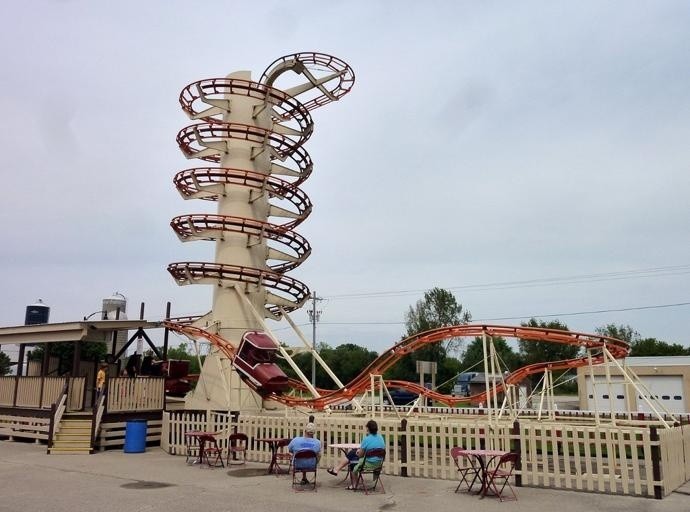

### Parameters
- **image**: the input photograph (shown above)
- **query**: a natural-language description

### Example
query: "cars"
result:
[383,382,439,405]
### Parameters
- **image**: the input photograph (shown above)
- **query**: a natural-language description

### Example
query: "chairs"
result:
[292,450,318,492]
[200,433,248,468]
[451,445,520,502]
[351,446,388,496]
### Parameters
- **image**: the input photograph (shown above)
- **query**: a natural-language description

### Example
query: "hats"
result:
[305,423,315,433]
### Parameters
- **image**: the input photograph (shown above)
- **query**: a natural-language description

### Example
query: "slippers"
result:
[344,484,356,491]
[327,467,338,476]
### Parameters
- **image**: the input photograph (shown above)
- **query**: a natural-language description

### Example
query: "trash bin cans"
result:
[124,419,147,453]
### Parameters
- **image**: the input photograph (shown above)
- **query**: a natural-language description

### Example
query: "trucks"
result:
[451,372,478,397]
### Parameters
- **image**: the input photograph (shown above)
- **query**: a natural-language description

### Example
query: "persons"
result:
[120,351,164,378]
[327,420,386,490]
[58,364,72,378]
[288,423,322,485]
[96,363,109,401]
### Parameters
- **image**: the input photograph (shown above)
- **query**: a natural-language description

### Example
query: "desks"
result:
[253,437,288,474]
[327,443,364,485]
[184,431,221,464]
